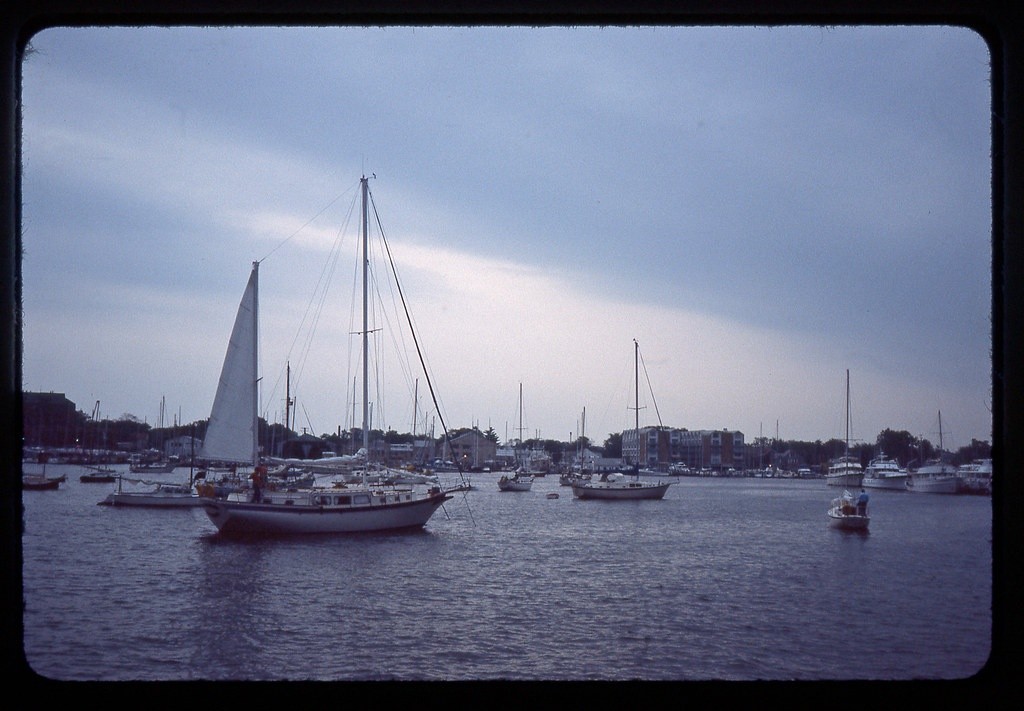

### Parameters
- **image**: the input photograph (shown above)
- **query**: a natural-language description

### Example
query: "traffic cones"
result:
[409,433,435,475]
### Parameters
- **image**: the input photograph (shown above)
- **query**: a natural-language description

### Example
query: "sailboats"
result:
[342,402,440,483]
[104,261,264,505]
[511,383,548,477]
[827,369,872,530]
[905,411,958,493]
[572,338,680,499]
[824,370,865,486]
[198,173,454,533]
[559,406,591,485]
[129,395,180,473]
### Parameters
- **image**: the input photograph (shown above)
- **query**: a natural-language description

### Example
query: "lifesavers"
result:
[196,482,216,498]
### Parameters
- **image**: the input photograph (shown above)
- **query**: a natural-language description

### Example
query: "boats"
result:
[22,473,67,490]
[956,471,990,490]
[80,415,115,483]
[861,452,907,490]
[267,464,309,476]
[127,454,142,464]
[296,466,351,474]
[497,474,535,491]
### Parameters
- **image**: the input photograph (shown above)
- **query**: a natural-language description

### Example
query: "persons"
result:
[250,467,262,503]
[856,489,869,516]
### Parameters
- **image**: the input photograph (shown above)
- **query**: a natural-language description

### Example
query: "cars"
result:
[669,462,782,474]
